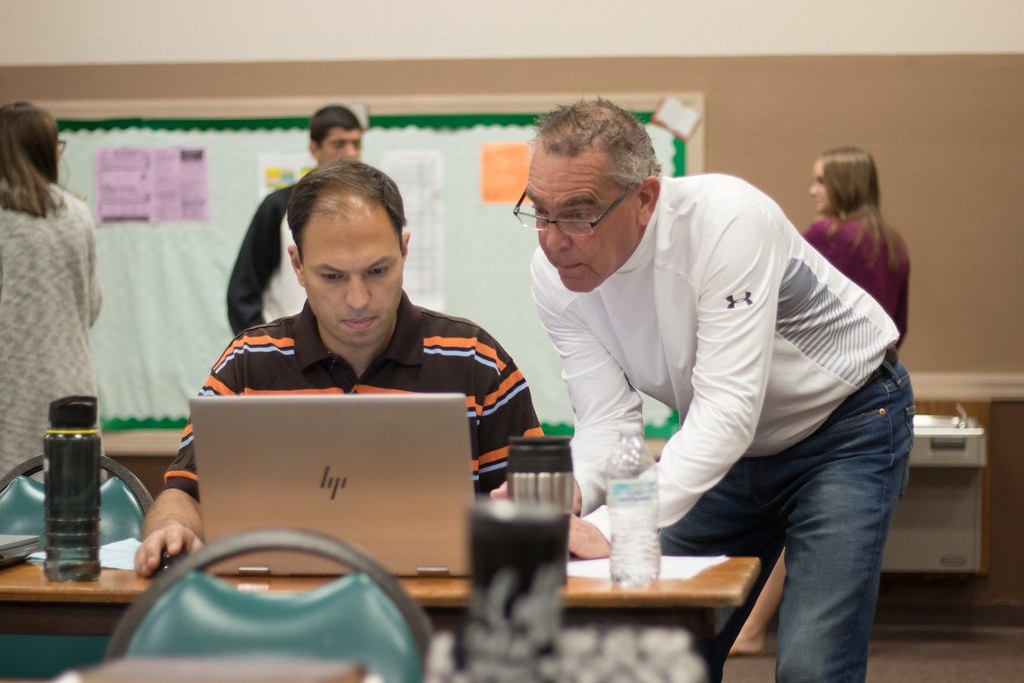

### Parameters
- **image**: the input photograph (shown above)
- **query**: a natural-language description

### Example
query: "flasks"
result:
[507,436,573,586]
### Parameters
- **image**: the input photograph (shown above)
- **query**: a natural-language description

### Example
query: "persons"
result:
[134,156,544,577]
[227,106,363,337]
[490,100,915,683]
[801,146,910,348]
[0,100,108,487]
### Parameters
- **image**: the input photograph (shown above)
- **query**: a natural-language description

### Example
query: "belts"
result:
[863,348,899,385]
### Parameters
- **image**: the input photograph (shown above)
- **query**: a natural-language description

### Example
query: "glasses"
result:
[56,139,66,155]
[513,180,637,237]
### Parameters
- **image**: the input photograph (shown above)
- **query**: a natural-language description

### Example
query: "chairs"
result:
[0,447,156,551]
[102,528,436,683]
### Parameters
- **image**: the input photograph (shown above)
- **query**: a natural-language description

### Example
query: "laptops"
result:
[188,392,477,578]
[0,534,40,569]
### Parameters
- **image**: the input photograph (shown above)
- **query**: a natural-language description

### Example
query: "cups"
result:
[465,504,566,683]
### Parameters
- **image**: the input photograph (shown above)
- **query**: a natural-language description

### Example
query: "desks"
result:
[93,426,187,505]
[0,556,765,641]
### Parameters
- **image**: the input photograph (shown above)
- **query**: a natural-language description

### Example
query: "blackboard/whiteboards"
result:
[51,111,689,437]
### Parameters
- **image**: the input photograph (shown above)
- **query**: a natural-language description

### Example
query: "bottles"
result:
[605,422,662,589]
[42,395,102,582]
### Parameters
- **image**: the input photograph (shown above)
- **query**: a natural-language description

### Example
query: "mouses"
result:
[151,545,188,579]
